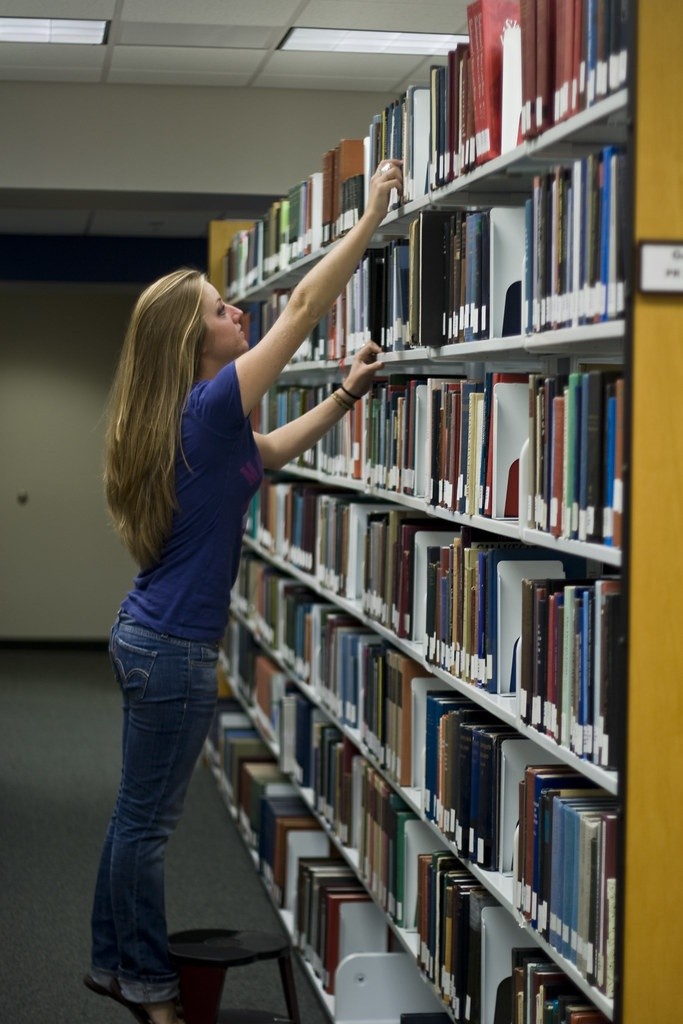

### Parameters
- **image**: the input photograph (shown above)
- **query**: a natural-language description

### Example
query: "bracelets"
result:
[340,385,361,401]
[331,393,353,412]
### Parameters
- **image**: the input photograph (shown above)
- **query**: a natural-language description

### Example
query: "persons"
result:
[80,154,406,1023]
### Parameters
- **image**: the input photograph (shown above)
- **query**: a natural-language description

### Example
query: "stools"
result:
[168,927,302,1024]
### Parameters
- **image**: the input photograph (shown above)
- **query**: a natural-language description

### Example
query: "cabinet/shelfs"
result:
[199,0,683,1023]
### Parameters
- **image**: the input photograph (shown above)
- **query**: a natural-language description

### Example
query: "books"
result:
[203,1,635,1024]
